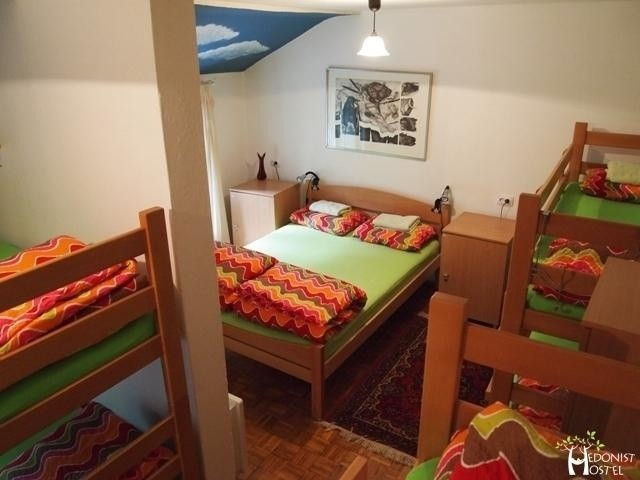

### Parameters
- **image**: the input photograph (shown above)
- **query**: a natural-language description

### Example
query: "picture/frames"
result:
[326,65,433,161]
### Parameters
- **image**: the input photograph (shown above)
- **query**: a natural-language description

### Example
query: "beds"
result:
[419,121,638,479]
[2,205,206,480]
[219,184,453,416]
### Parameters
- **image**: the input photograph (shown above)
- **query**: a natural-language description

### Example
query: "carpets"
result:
[225,279,493,468]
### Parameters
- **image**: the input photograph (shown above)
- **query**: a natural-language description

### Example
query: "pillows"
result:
[288,203,436,253]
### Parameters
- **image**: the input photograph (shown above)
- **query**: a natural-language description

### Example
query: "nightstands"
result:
[228,176,301,248]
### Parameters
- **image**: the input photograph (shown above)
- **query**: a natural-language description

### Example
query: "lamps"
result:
[357,0,392,57]
[296,170,319,193]
